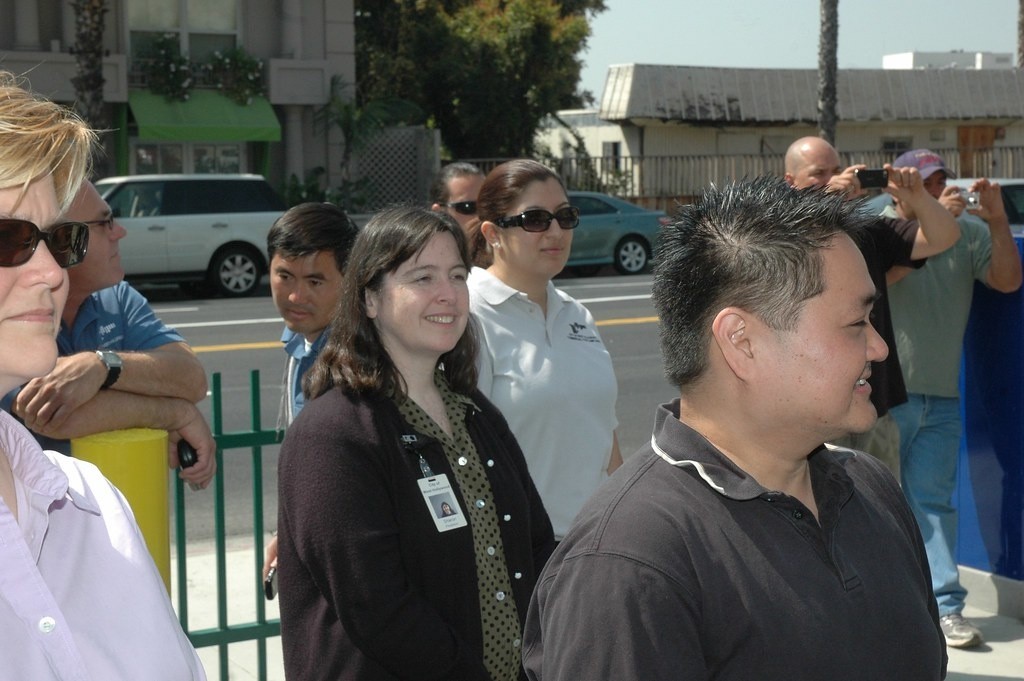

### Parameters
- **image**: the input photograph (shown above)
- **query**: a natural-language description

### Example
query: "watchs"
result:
[97,348,124,389]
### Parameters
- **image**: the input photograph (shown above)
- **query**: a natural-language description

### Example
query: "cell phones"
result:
[856,169,889,189]
[265,568,277,600]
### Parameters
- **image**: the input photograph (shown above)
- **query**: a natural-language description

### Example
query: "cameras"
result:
[956,190,981,211]
[174,440,202,490]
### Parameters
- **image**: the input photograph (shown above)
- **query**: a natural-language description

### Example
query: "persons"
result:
[0,156,623,590]
[785,137,1022,646]
[522,178,949,681]
[441,500,458,518]
[280,202,555,681]
[0,82,204,681]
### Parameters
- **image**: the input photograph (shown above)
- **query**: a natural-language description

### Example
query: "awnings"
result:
[127,84,283,142]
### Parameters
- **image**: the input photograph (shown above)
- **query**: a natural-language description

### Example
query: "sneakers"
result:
[939,614,983,647]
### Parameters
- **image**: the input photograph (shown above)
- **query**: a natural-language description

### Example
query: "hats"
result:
[893,149,957,182]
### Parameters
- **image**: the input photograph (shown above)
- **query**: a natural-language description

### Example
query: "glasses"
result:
[0,218,89,268]
[494,207,580,232]
[81,214,114,230]
[438,201,477,215]
